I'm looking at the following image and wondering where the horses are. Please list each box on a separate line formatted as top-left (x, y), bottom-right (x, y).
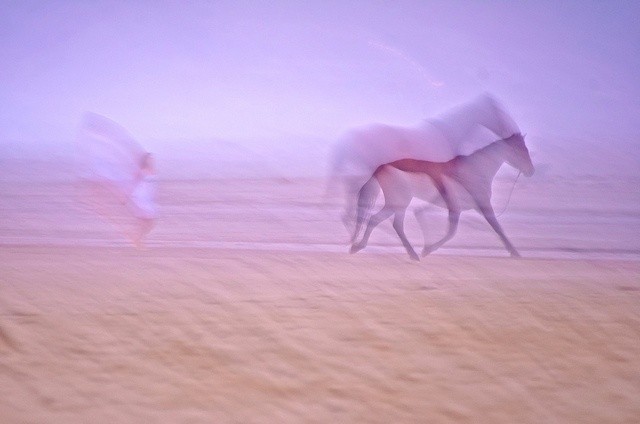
top-left (324, 93), bottom-right (521, 246)
top-left (347, 132), bottom-right (535, 261)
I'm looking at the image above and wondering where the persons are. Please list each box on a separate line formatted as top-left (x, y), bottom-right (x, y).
top-left (128, 152), bottom-right (164, 247)
